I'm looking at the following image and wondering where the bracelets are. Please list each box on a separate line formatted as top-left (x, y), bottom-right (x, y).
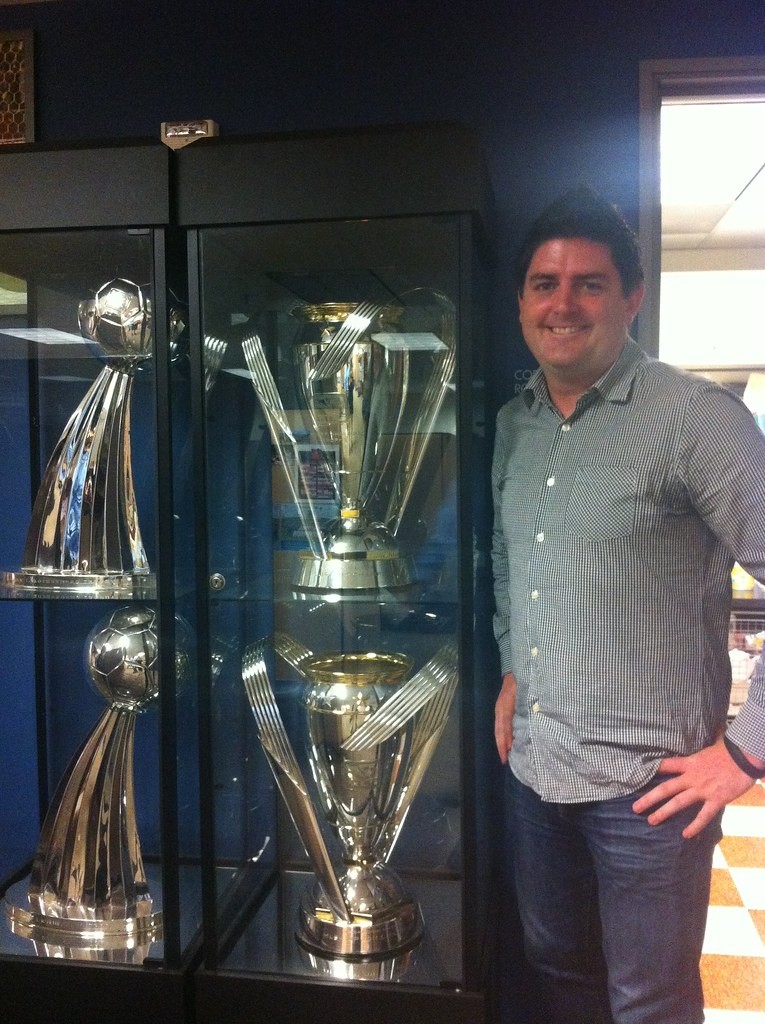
top-left (722, 735), bottom-right (765, 781)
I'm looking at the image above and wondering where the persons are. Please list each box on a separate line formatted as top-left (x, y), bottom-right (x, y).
top-left (492, 189), bottom-right (765, 1023)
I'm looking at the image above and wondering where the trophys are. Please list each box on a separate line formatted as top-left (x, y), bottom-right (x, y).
top-left (3, 274), bottom-right (164, 593)
top-left (242, 633), bottom-right (462, 963)
top-left (240, 287), bottom-right (462, 593)
top-left (3, 603), bottom-right (167, 934)
